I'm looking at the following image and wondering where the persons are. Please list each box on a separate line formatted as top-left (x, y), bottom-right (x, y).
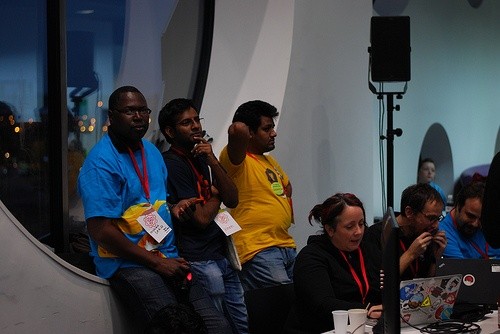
top-left (77, 85), bottom-right (203, 334)
top-left (158, 101), bottom-right (251, 334)
top-left (218, 99), bottom-right (297, 285)
top-left (419, 158), bottom-right (448, 204)
top-left (289, 153), bottom-right (500, 334)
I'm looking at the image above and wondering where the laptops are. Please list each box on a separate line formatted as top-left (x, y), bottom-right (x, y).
top-left (435, 258), bottom-right (500, 306)
top-left (364, 274), bottom-right (463, 328)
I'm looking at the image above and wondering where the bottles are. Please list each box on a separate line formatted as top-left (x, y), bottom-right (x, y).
top-left (446, 194), bottom-right (455, 215)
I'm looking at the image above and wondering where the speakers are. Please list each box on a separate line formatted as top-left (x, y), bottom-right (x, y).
top-left (369, 15), bottom-right (411, 82)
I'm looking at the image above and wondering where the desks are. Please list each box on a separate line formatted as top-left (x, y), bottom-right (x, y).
top-left (322, 309), bottom-right (500, 334)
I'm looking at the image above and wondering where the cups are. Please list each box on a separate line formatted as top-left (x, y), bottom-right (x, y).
top-left (349, 309), bottom-right (368, 334)
top-left (332, 310), bottom-right (349, 334)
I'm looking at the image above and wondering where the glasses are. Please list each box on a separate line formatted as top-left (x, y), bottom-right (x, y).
top-left (180, 118), bottom-right (205, 127)
top-left (115, 108), bottom-right (151, 116)
top-left (420, 211), bottom-right (446, 223)
